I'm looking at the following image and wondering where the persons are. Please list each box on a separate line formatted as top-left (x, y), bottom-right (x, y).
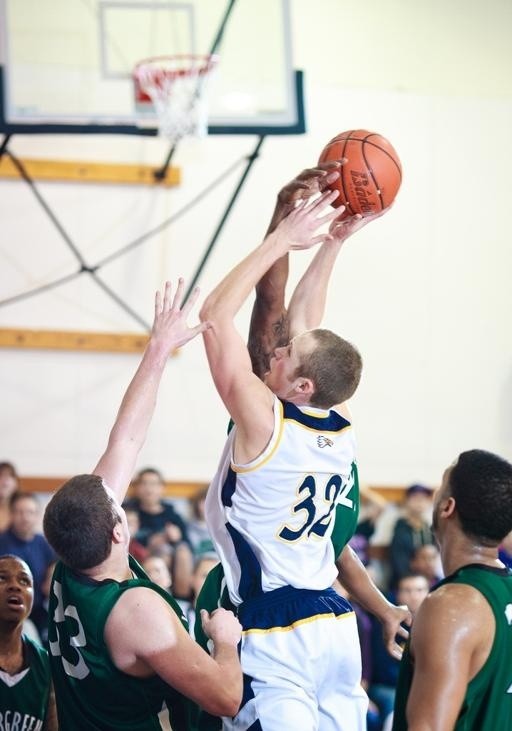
top-left (164, 158), bottom-right (416, 731)
top-left (199, 187), bottom-right (399, 730)
top-left (2, 446), bottom-right (512, 731)
top-left (42, 276), bottom-right (246, 731)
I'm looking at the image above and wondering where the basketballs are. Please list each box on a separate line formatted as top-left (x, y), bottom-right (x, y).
top-left (317, 129), bottom-right (403, 219)
